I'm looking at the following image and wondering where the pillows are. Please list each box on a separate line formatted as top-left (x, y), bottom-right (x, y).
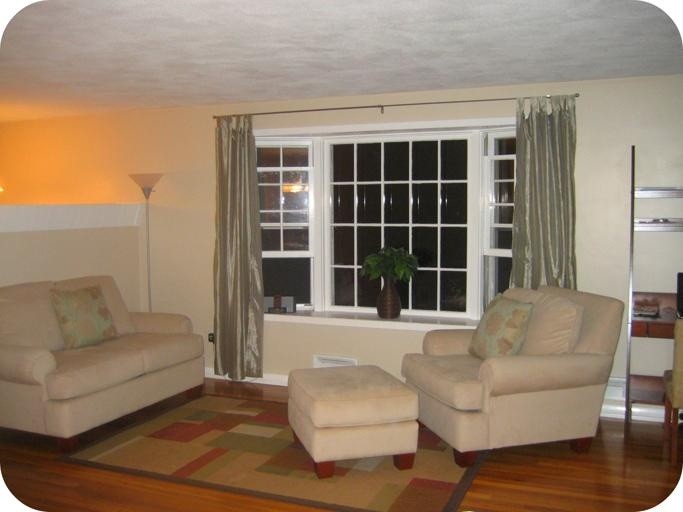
top-left (51, 284), bottom-right (120, 350)
top-left (468, 293), bottom-right (534, 363)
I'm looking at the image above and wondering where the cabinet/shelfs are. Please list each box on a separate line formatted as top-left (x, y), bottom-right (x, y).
top-left (624, 142), bottom-right (683, 437)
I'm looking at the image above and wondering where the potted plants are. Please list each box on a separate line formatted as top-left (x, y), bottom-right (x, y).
top-left (362, 251), bottom-right (419, 318)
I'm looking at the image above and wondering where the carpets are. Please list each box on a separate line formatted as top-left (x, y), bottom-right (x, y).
top-left (71, 394), bottom-right (486, 511)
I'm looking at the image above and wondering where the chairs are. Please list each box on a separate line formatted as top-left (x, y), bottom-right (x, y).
top-left (401, 285), bottom-right (626, 468)
top-left (663, 321), bottom-right (683, 462)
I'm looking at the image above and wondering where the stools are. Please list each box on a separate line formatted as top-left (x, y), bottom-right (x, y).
top-left (285, 365), bottom-right (417, 476)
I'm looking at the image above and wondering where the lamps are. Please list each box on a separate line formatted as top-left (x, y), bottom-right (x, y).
top-left (129, 172), bottom-right (162, 311)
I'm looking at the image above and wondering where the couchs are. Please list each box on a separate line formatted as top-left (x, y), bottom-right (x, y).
top-left (2, 276), bottom-right (206, 465)
